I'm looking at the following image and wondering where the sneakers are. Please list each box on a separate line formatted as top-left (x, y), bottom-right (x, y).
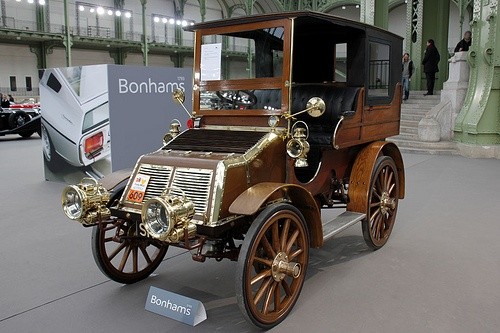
top-left (403, 95), bottom-right (409, 100)
top-left (423, 91), bottom-right (434, 95)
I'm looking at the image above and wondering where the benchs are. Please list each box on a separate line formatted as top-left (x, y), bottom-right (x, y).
top-left (246, 83), bottom-right (365, 149)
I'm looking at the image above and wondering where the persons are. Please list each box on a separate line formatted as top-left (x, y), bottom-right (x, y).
top-left (454, 31), bottom-right (472, 52)
top-left (0, 93), bottom-right (14, 108)
top-left (422, 39), bottom-right (440, 95)
top-left (402, 53), bottom-right (413, 101)
